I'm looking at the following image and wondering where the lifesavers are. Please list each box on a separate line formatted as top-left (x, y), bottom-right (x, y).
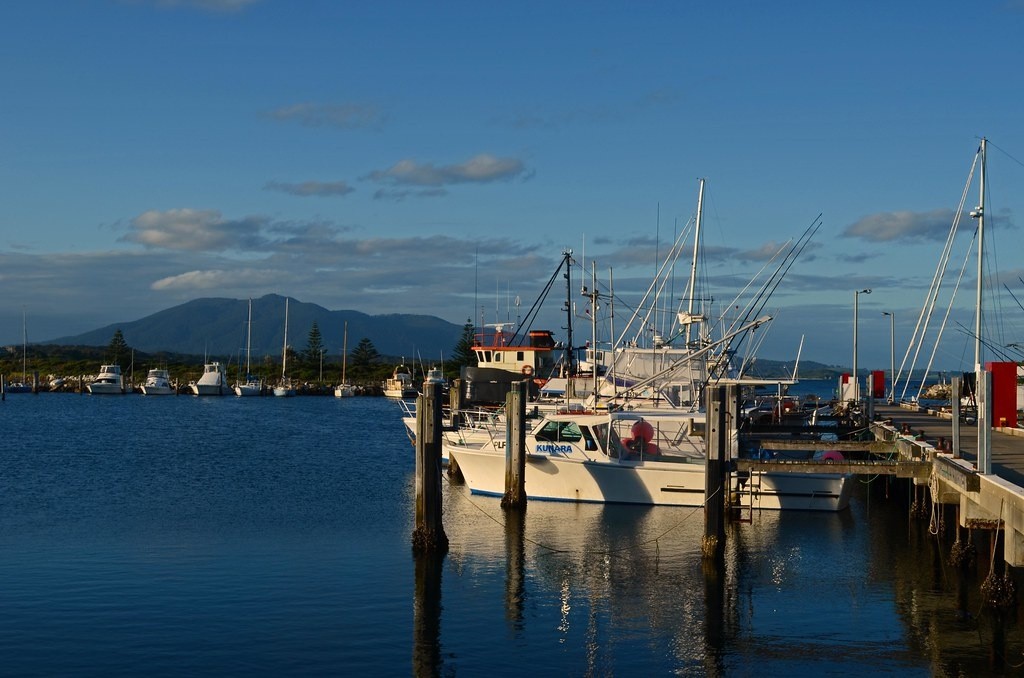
top-left (522, 365), bottom-right (534, 375)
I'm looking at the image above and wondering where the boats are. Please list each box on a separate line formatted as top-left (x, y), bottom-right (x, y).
top-left (90, 364), bottom-right (124, 393)
top-left (140, 369), bottom-right (175, 395)
top-left (194, 363), bottom-right (229, 394)
top-left (382, 356), bottom-right (419, 399)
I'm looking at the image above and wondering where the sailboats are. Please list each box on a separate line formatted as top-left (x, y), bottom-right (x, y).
top-left (401, 129), bottom-right (1024, 513)
top-left (331, 320), bottom-right (357, 398)
top-left (234, 297), bottom-right (268, 396)
top-left (274, 298), bottom-right (297, 397)
